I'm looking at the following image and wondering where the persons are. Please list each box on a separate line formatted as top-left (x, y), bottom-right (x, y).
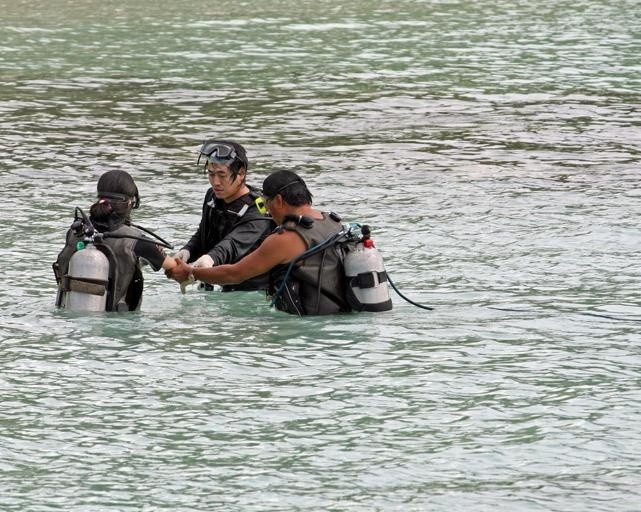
top-left (53, 169), bottom-right (179, 311)
top-left (164, 141), bottom-right (276, 292)
top-left (164, 169), bottom-right (352, 315)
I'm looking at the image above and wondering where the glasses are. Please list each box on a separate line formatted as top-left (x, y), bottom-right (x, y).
top-left (199, 141), bottom-right (244, 164)
top-left (131, 195), bottom-right (141, 210)
top-left (255, 195), bottom-right (270, 218)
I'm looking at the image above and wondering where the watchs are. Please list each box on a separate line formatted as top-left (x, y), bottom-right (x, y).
top-left (187, 266), bottom-right (197, 282)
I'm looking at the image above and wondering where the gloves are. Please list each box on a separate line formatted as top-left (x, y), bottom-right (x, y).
top-left (186, 253), bottom-right (215, 286)
top-left (164, 248), bottom-right (191, 279)
